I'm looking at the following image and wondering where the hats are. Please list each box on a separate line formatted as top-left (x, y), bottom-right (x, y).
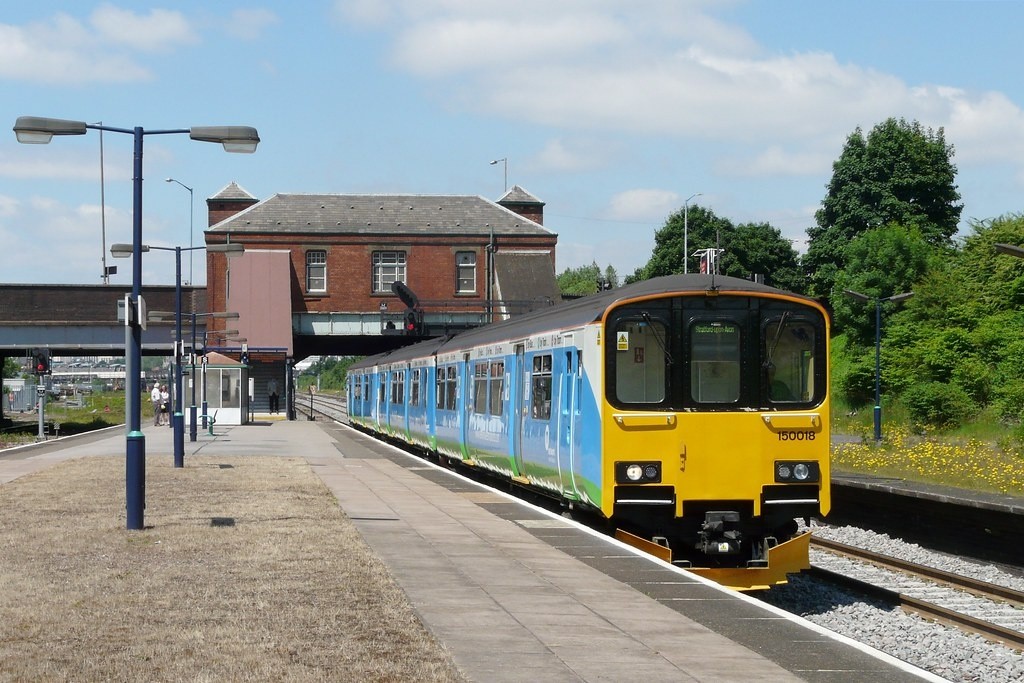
top-left (154, 383), bottom-right (160, 388)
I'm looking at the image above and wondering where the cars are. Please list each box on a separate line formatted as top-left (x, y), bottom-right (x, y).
top-left (23, 359), bottom-right (146, 403)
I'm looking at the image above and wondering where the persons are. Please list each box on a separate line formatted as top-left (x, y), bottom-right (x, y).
top-left (160, 385), bottom-right (170, 425)
top-left (151, 383), bottom-right (162, 426)
top-left (769, 362), bottom-right (794, 400)
top-left (268, 376), bottom-right (280, 415)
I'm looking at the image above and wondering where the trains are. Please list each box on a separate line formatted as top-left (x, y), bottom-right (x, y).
top-left (346, 269), bottom-right (832, 594)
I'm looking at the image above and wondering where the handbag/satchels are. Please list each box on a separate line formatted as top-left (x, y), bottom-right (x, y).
top-left (161, 405), bottom-right (166, 409)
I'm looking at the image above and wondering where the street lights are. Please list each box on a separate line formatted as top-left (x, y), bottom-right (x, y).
top-left (490, 158), bottom-right (508, 194)
top-left (847, 290), bottom-right (916, 441)
top-left (12, 115), bottom-right (262, 530)
top-left (148, 311), bottom-right (242, 442)
top-left (109, 243), bottom-right (247, 469)
top-left (191, 335), bottom-right (248, 355)
top-left (166, 177), bottom-right (194, 285)
top-left (683, 193), bottom-right (706, 274)
top-left (170, 329), bottom-right (240, 432)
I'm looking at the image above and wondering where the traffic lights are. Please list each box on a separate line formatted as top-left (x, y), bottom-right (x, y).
top-left (404, 309), bottom-right (420, 337)
top-left (32, 347), bottom-right (50, 374)
